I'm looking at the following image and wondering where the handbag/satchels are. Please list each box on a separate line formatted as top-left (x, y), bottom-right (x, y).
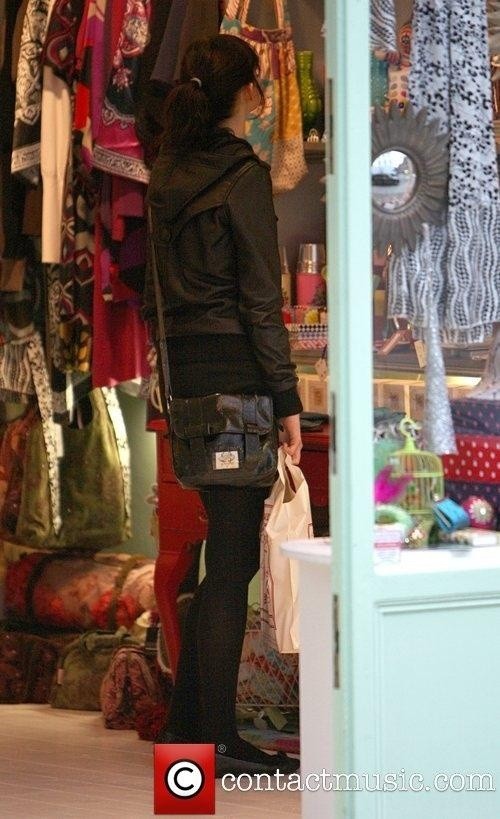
top-left (1, 619), bottom-right (170, 728)
top-left (2, 408), bottom-right (132, 560)
top-left (216, 17), bottom-right (310, 195)
top-left (164, 394), bottom-right (278, 489)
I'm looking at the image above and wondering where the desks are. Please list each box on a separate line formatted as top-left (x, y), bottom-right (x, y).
top-left (279, 528), bottom-right (500, 819)
top-left (146, 418), bottom-right (328, 686)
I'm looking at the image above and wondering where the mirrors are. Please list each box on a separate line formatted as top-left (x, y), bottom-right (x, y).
top-left (372, 98), bottom-right (450, 258)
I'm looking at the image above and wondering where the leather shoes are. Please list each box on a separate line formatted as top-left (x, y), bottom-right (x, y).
top-left (153, 731), bottom-right (301, 779)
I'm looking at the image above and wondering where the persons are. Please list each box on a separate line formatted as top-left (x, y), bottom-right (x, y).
top-left (144, 34), bottom-right (303, 777)
